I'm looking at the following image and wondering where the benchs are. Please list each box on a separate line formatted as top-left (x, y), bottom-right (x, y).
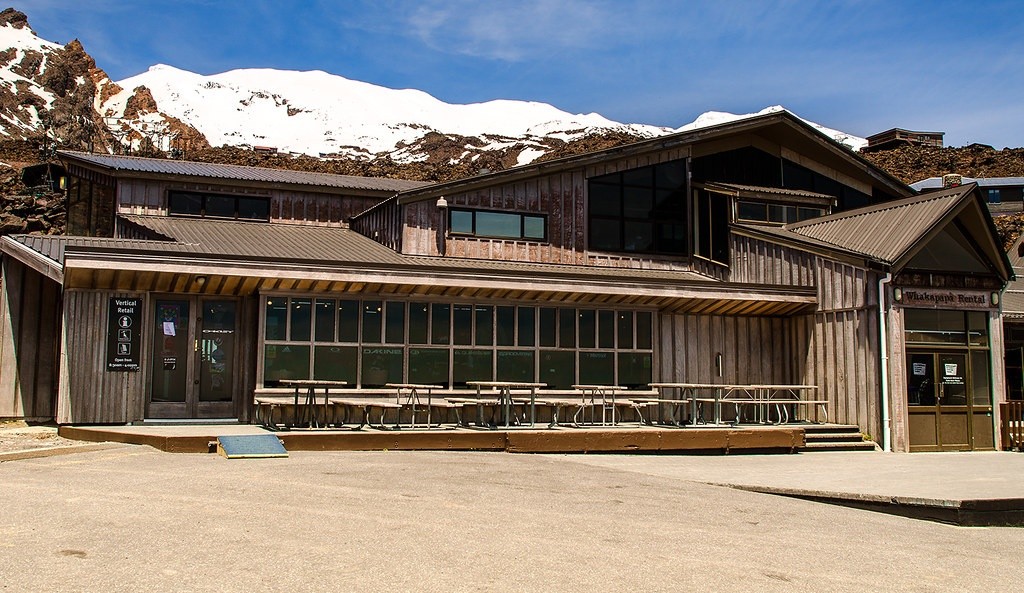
top-left (254, 395), bottom-right (830, 427)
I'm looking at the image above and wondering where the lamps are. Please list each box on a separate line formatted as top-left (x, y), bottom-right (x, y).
top-left (893, 287), bottom-right (902, 301)
top-left (991, 292), bottom-right (999, 305)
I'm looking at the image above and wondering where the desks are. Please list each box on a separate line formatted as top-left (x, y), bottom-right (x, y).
top-left (279, 379), bottom-right (347, 432)
top-left (386, 383), bottom-right (442, 429)
top-left (752, 384), bottom-right (819, 423)
top-left (647, 382), bottom-right (718, 429)
top-left (467, 381), bottom-right (547, 427)
top-left (705, 383), bottom-right (755, 422)
top-left (573, 385), bottom-right (627, 427)
